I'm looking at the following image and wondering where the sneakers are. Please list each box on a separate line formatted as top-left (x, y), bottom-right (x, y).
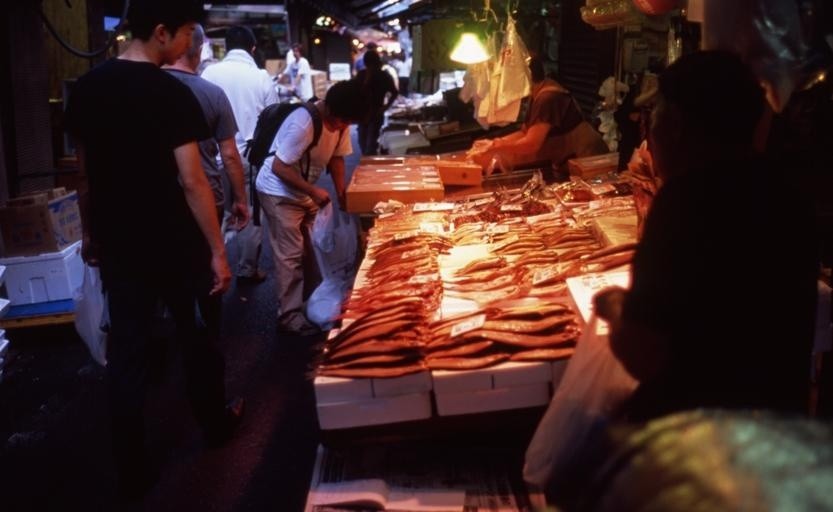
top-left (278, 311), bottom-right (321, 336)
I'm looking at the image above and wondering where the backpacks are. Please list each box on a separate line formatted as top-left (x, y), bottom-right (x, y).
top-left (248, 102), bottom-right (344, 168)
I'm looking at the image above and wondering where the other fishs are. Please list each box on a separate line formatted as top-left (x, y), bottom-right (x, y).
top-left (305, 212), bottom-right (639, 378)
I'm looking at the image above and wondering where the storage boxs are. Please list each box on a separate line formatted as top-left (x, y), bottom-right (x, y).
top-left (1, 190), bottom-right (83, 257)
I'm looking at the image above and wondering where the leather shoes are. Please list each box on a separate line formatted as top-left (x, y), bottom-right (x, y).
top-left (237, 272), bottom-right (267, 288)
top-left (205, 397), bottom-right (246, 447)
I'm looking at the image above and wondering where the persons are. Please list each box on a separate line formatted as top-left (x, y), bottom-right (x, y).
top-left (471, 50), bottom-right (609, 179)
top-left (254, 77), bottom-right (367, 338)
top-left (348, 41), bottom-right (412, 98)
top-left (274, 40), bottom-right (315, 101)
top-left (203, 25), bottom-right (282, 288)
top-left (352, 51), bottom-right (399, 157)
top-left (592, 49), bottom-right (819, 419)
top-left (60, 1), bottom-right (247, 502)
top-left (159, 20), bottom-right (252, 236)
